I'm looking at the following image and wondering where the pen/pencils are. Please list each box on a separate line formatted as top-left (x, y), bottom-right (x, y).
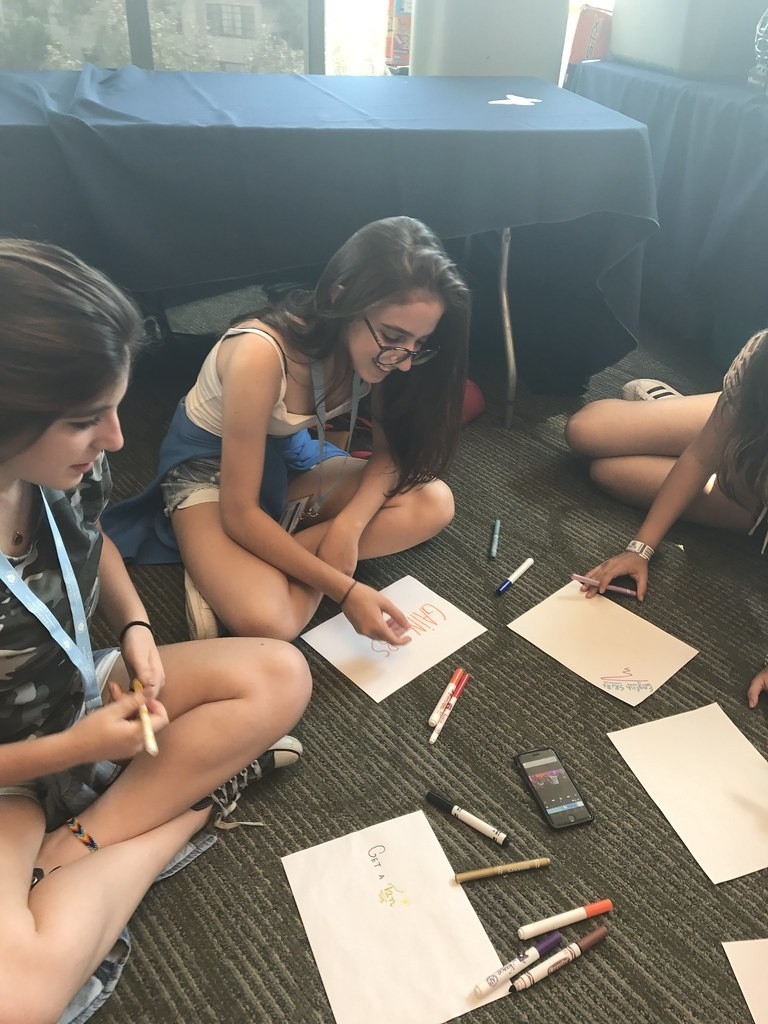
top-left (490, 519), bottom-right (500, 558)
top-left (133, 679), bottom-right (159, 755)
top-left (429, 673), bottom-right (470, 745)
top-left (455, 858), bottom-right (551, 883)
top-left (571, 574), bottom-right (636, 597)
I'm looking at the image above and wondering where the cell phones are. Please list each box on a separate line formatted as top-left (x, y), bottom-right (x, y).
top-left (515, 748), bottom-right (593, 830)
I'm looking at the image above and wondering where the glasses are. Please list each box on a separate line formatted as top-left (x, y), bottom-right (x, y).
top-left (361, 311), bottom-right (441, 366)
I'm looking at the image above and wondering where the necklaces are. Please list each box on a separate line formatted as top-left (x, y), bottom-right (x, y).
top-left (1, 492), bottom-right (33, 545)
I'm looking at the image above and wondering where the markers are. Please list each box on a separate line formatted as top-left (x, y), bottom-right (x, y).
top-left (475, 932), bottom-right (562, 997)
top-left (509, 925), bottom-right (609, 994)
top-left (427, 668), bottom-right (463, 727)
top-left (497, 557), bottom-right (534, 592)
top-left (426, 791), bottom-right (510, 846)
top-left (517, 897), bottom-right (614, 943)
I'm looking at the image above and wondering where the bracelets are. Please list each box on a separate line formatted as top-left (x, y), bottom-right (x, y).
top-left (118, 620), bottom-right (155, 641)
top-left (336, 580), bottom-right (357, 607)
top-left (625, 539), bottom-right (654, 560)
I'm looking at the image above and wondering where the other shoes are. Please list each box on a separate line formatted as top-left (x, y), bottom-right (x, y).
top-left (184, 567), bottom-right (218, 641)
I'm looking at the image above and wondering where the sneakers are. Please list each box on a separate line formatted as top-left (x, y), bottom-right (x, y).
top-left (622, 378), bottom-right (685, 401)
top-left (29, 865), bottom-right (61, 891)
top-left (189, 734), bottom-right (303, 830)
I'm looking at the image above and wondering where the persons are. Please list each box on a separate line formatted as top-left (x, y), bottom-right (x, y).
top-left (98, 215), bottom-right (471, 641)
top-left (0, 236), bottom-right (311, 1024)
top-left (566, 330), bottom-right (768, 708)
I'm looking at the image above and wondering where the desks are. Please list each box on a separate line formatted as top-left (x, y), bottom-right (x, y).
top-left (567, 56), bottom-right (766, 383)
top-left (0, 65), bottom-right (659, 427)
